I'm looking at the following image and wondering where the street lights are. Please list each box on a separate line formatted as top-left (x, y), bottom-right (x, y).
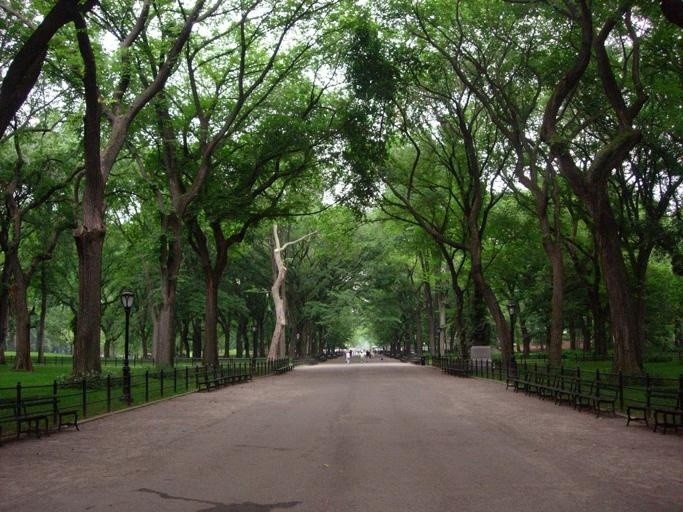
top-left (507, 303), bottom-right (515, 383)
top-left (119, 290), bottom-right (135, 402)
top-left (250, 325), bottom-right (258, 370)
top-left (436, 325), bottom-right (442, 359)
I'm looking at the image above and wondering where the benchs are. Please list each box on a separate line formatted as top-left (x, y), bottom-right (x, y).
top-left (195, 357), bottom-right (293, 392)
top-left (444, 359), bottom-right (469, 378)
top-left (504, 368), bottom-right (682, 434)
top-left (2, 398), bottom-right (80, 440)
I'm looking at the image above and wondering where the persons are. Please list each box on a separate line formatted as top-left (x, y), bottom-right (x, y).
top-left (345, 349), bottom-right (371, 364)
